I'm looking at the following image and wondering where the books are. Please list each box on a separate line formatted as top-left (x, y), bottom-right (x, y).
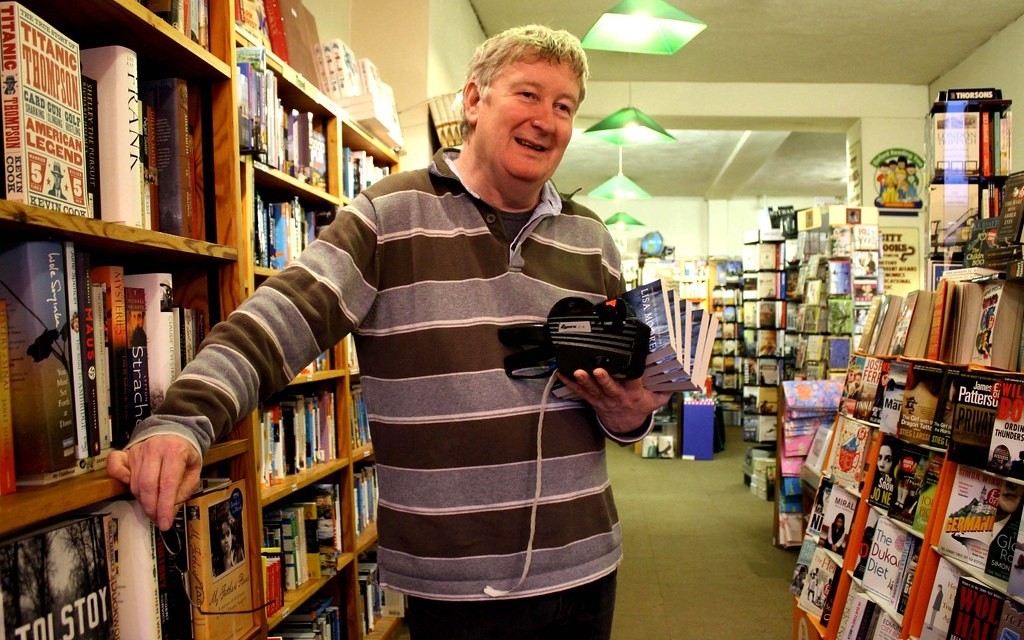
top-left (641, 206), bottom-right (880, 548)
top-left (789, 87), bottom-right (1024, 640)
top-left (549, 279), bottom-right (719, 400)
top-left (428, 90), bottom-right (467, 148)
top-left (0, 0), bottom-right (406, 640)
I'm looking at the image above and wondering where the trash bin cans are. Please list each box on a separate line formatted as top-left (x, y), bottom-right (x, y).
top-left (681, 399), bottom-right (717, 460)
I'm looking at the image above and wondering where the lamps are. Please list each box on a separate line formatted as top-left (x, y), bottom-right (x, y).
top-left (584, 106), bottom-right (677, 146)
top-left (587, 174), bottom-right (653, 231)
top-left (581, 0), bottom-right (708, 56)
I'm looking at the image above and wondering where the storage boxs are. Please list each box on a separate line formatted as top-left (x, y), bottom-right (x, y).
top-left (635, 435), bottom-right (675, 458)
top-left (682, 401), bottom-right (715, 458)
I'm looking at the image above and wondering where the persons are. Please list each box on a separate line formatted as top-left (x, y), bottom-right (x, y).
top-left (105, 24), bottom-right (671, 640)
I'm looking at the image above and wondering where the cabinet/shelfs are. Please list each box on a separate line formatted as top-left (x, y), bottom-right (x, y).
top-left (641, 87), bottom-right (1024, 640)
top-left (0, 0), bottom-right (407, 640)
